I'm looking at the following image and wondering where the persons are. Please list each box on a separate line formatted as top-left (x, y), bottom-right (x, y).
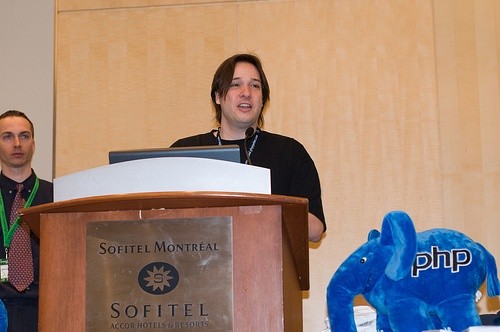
top-left (1, 110), bottom-right (55, 332)
top-left (168, 54), bottom-right (328, 244)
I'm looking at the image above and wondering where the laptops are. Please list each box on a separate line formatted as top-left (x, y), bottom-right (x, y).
top-left (108, 145), bottom-right (241, 164)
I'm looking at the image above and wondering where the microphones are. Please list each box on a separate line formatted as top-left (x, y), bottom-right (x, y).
top-left (243, 127), bottom-right (255, 165)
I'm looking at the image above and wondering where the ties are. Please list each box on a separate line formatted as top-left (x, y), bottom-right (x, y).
top-left (6, 184), bottom-right (34, 292)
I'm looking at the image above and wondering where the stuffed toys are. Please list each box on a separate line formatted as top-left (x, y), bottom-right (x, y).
top-left (325, 208), bottom-right (500, 332)
